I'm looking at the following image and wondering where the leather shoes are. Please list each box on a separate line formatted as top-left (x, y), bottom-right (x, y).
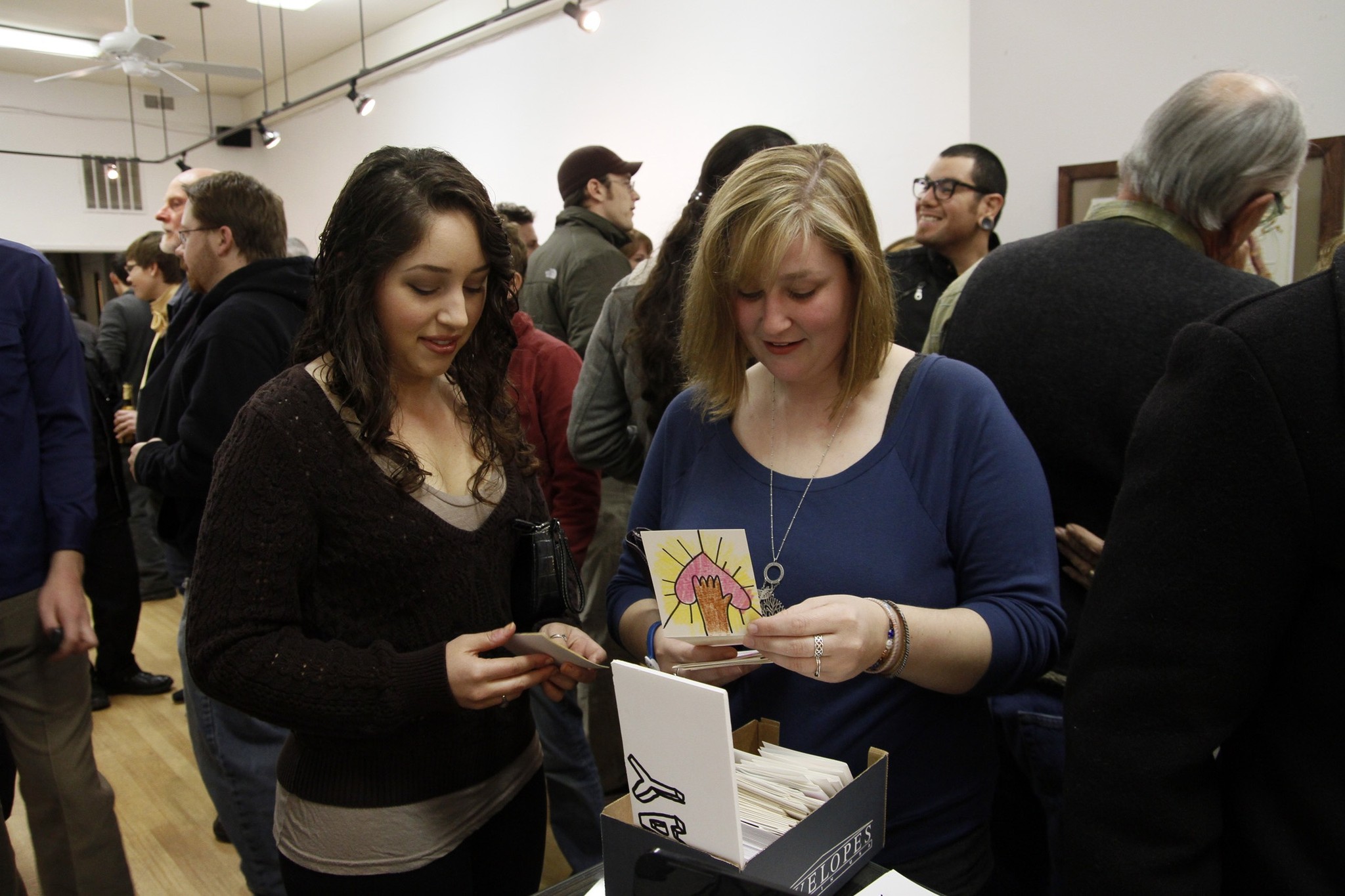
top-left (88, 670), bottom-right (173, 711)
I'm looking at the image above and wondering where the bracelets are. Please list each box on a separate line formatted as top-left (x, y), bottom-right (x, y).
top-left (863, 597), bottom-right (911, 680)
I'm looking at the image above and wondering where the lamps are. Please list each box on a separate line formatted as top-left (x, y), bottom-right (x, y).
top-left (176, 159), bottom-right (191, 172)
top-left (346, 89), bottom-right (375, 116)
top-left (563, 2), bottom-right (602, 33)
top-left (106, 164), bottom-right (119, 179)
top-left (257, 128), bottom-right (281, 151)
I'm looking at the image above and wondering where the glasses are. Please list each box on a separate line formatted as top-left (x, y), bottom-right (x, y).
top-left (608, 177), bottom-right (635, 194)
top-left (178, 227), bottom-right (208, 245)
top-left (124, 264), bottom-right (138, 272)
top-left (912, 175), bottom-right (984, 201)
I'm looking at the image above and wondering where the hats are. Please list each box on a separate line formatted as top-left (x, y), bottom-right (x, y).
top-left (556, 146), bottom-right (643, 201)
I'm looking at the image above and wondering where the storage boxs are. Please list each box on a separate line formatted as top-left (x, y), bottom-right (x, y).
top-left (599, 717), bottom-right (889, 896)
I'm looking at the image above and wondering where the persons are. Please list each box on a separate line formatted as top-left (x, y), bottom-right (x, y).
top-left (112, 230), bottom-right (187, 445)
top-left (570, 125), bottom-right (796, 485)
top-left (879, 145), bottom-right (1007, 352)
top-left (491, 203), bottom-right (538, 262)
top-left (58, 277), bottom-right (172, 711)
top-left (96, 258), bottom-right (156, 382)
top-left (619, 228), bottom-right (655, 269)
top-left (132, 170), bottom-right (321, 843)
top-left (1139, 245), bottom-right (1344, 894)
top-left (930, 66), bottom-right (1308, 689)
top-left (490, 213), bottom-right (615, 878)
top-left (2, 239), bottom-right (137, 896)
top-left (134, 168), bottom-right (220, 442)
top-left (64, 296), bottom-right (96, 366)
top-left (609, 143), bottom-right (1064, 890)
top-left (518, 144), bottom-right (653, 800)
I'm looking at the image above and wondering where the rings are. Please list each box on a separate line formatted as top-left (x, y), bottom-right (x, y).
top-left (814, 656), bottom-right (820, 678)
top-left (502, 696), bottom-right (507, 703)
top-left (196, 144), bottom-right (606, 896)
top-left (815, 634), bottom-right (823, 660)
top-left (550, 634), bottom-right (567, 642)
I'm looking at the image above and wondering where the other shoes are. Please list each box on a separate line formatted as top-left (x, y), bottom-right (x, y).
top-left (137, 580), bottom-right (179, 602)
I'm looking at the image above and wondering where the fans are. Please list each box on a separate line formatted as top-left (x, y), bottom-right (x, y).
top-left (33, 1), bottom-right (264, 96)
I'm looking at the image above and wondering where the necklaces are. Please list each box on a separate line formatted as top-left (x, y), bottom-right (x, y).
top-left (763, 375), bottom-right (854, 583)
